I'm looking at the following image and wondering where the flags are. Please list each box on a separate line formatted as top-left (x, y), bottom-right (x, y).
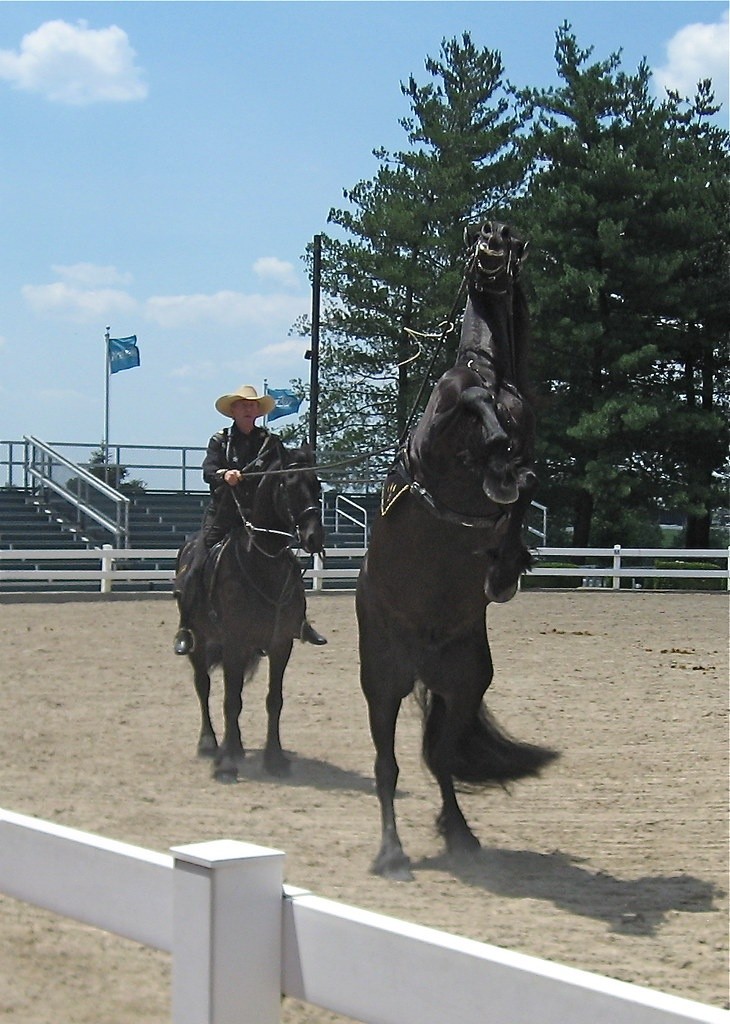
top-left (109, 336), bottom-right (140, 374)
top-left (267, 388), bottom-right (304, 420)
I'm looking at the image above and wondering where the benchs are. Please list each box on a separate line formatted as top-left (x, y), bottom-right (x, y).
top-left (0, 482), bottom-right (382, 591)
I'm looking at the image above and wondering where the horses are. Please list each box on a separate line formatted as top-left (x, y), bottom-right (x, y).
top-left (353, 222), bottom-right (566, 882)
top-left (170, 432), bottom-right (327, 785)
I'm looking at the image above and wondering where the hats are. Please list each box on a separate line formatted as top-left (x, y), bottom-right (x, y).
top-left (214, 386), bottom-right (276, 419)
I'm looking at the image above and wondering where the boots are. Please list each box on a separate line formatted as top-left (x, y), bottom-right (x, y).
top-left (296, 620), bottom-right (328, 646)
top-left (173, 590), bottom-right (195, 654)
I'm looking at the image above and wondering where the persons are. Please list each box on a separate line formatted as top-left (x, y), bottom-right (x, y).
top-left (174, 385), bottom-right (328, 655)
